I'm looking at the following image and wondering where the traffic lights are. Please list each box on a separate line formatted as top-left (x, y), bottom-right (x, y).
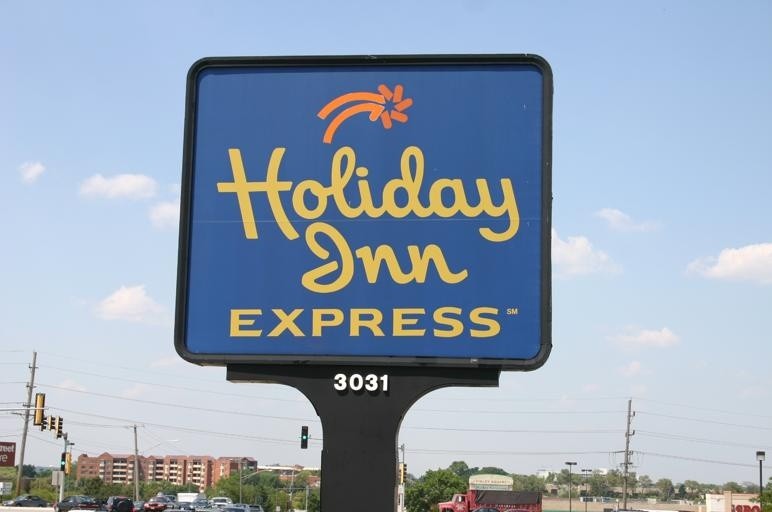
top-left (300, 425), bottom-right (309, 450)
top-left (60, 453), bottom-right (66, 471)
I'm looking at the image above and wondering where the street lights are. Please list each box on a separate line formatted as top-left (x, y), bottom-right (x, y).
top-left (581, 469), bottom-right (592, 512)
top-left (755, 450), bottom-right (766, 508)
top-left (565, 461), bottom-right (577, 511)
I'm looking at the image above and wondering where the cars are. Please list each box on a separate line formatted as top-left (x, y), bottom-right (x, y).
top-left (102, 495), bottom-right (135, 512)
top-left (134, 492), bottom-right (265, 512)
top-left (2, 494), bottom-right (48, 507)
top-left (53, 495), bottom-right (101, 512)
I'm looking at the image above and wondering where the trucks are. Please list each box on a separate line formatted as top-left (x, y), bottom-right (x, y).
top-left (438, 489), bottom-right (543, 512)
